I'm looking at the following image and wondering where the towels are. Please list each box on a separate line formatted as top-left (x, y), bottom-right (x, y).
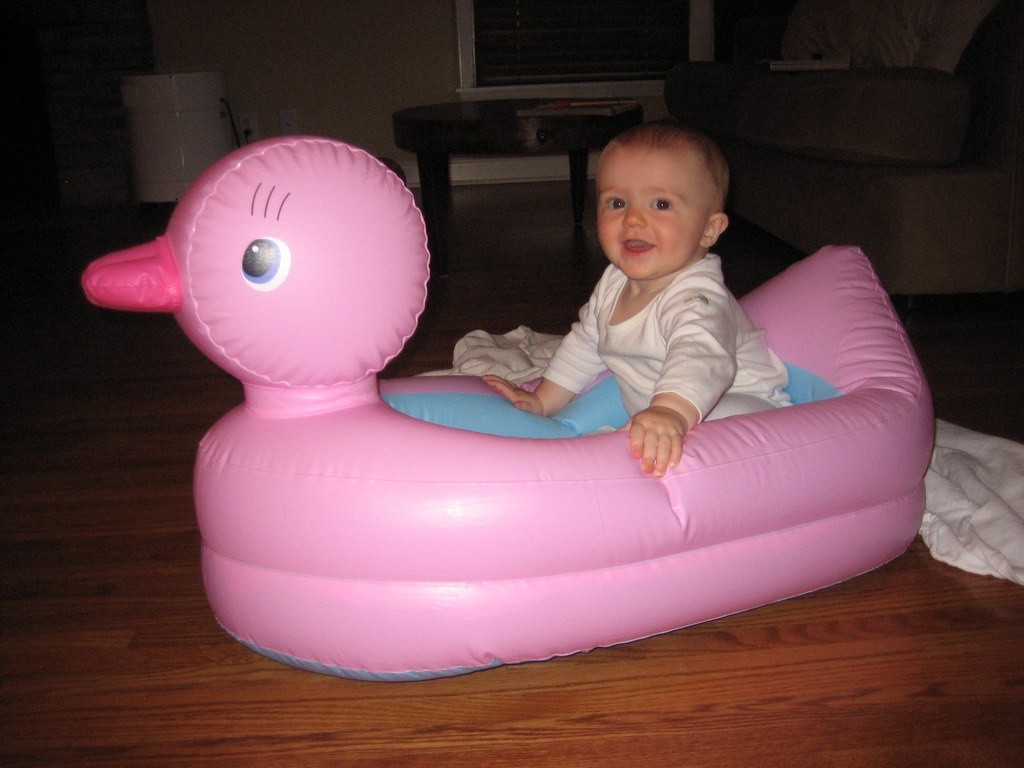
top-left (414, 324), bottom-right (569, 389)
top-left (918, 418), bottom-right (1024, 586)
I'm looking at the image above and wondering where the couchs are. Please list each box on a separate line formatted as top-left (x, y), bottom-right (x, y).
top-left (659, 0), bottom-right (1024, 312)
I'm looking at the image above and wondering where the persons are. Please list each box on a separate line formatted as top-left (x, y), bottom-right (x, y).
top-left (480, 117), bottom-right (794, 477)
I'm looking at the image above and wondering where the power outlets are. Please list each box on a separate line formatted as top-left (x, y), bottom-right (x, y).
top-left (279, 112), bottom-right (296, 135)
top-left (238, 113), bottom-right (258, 141)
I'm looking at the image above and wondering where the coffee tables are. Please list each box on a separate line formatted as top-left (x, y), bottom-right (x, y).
top-left (392, 95), bottom-right (641, 273)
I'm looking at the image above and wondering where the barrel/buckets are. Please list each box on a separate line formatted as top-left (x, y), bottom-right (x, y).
top-left (120, 67), bottom-right (239, 207)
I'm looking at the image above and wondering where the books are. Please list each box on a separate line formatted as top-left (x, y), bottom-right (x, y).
top-left (771, 55), bottom-right (873, 70)
top-left (516, 99), bottom-right (638, 117)
